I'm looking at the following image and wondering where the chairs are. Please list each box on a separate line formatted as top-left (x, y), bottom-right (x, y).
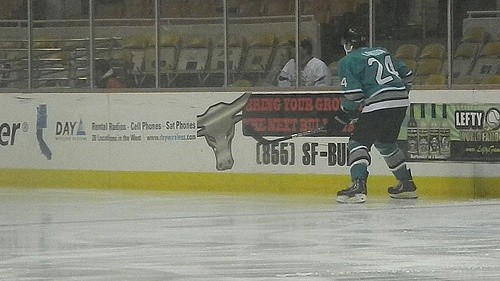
top-left (0, 29), bottom-right (316, 86)
top-left (397, 27), bottom-right (500, 85)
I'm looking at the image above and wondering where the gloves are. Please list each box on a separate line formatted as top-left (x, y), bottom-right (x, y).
top-left (325, 108), bottom-right (348, 134)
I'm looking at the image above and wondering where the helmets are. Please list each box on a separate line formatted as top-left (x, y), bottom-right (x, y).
top-left (340, 27), bottom-right (366, 55)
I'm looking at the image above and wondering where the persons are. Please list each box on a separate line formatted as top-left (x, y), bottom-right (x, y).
top-left (278, 38), bottom-right (332, 87)
top-left (324, 27), bottom-right (418, 203)
top-left (93, 59), bottom-right (121, 88)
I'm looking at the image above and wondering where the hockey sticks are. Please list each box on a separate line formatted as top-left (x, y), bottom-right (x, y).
top-left (244, 123), bottom-right (326, 145)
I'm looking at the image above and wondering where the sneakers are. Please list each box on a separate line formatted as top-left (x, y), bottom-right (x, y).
top-left (336, 178), bottom-right (368, 204)
top-left (387, 180), bottom-right (418, 198)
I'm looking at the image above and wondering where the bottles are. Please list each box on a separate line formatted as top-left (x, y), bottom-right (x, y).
top-left (429, 104), bottom-right (440, 160)
top-left (440, 104), bottom-right (451, 159)
top-left (406, 103), bottom-right (419, 160)
top-left (417, 103), bottom-right (430, 160)
